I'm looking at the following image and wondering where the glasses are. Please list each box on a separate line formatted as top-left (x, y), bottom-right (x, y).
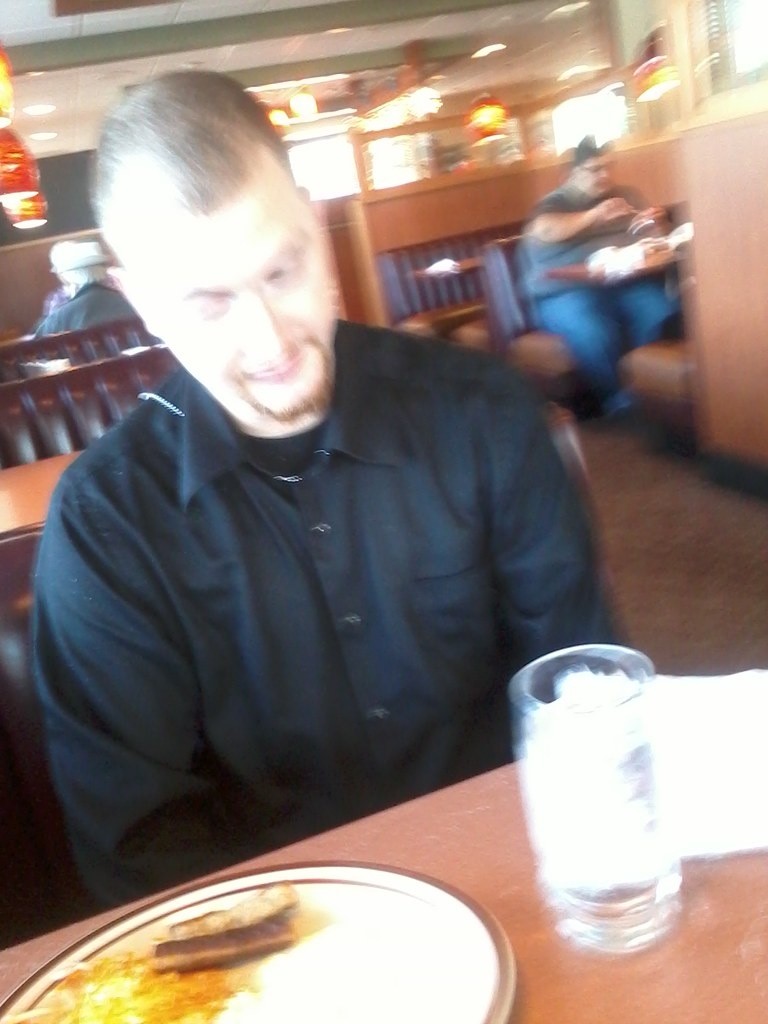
top-left (581, 164), bottom-right (604, 172)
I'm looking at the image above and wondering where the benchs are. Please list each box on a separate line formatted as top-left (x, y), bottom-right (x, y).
top-left (378, 238), bottom-right (696, 440)
top-left (0, 317), bottom-right (184, 953)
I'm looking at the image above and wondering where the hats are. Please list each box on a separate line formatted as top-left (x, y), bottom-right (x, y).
top-left (55, 241), bottom-right (110, 273)
top-left (575, 135), bottom-right (616, 164)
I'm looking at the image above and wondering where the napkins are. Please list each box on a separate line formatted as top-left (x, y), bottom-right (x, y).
top-left (563, 668), bottom-right (768, 861)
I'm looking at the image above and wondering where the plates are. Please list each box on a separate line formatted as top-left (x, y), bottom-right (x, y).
top-left (0, 860), bottom-right (515, 1024)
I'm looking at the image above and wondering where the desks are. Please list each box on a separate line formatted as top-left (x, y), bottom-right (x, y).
top-left (548, 244), bottom-right (678, 289)
top-left (414, 254), bottom-right (483, 277)
top-left (0, 762), bottom-right (768, 1024)
top-left (0, 451), bottom-right (86, 539)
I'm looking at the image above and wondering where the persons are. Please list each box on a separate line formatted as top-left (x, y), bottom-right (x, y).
top-left (30, 71), bottom-right (617, 918)
top-left (32, 239), bottom-right (146, 369)
top-left (518, 143), bottom-right (681, 418)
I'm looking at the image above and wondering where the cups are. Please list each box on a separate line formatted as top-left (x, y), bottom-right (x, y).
top-left (507, 642), bottom-right (685, 958)
